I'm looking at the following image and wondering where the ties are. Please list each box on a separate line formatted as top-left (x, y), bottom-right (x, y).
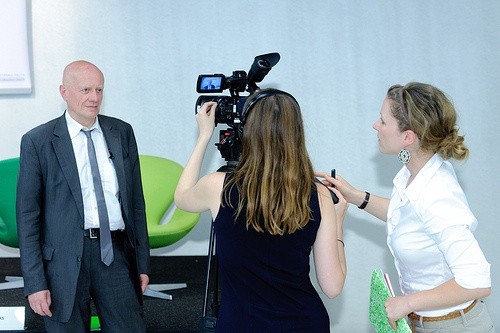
top-left (81, 128), bottom-right (114, 266)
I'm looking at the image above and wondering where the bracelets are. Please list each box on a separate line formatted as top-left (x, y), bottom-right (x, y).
top-left (358, 191), bottom-right (370, 209)
top-left (337, 238), bottom-right (346, 248)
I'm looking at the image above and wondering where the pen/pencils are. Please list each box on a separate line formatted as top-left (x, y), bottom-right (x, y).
top-left (330, 169), bottom-right (336, 189)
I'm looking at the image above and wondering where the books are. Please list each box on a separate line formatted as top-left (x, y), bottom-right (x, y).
top-left (369, 270), bottom-right (412, 333)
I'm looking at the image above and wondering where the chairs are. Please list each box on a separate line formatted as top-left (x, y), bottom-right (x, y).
top-left (0, 155), bottom-right (200, 300)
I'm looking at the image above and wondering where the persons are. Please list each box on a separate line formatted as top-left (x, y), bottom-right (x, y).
top-left (15, 60), bottom-right (150, 333)
top-left (204, 79), bottom-right (215, 90)
top-left (174, 88), bottom-right (349, 333)
top-left (314, 81), bottom-right (497, 333)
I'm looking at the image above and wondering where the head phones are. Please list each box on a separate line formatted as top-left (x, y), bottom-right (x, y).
top-left (237, 89), bottom-right (300, 140)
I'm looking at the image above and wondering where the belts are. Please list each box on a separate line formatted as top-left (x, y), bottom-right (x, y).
top-left (409, 299), bottom-right (478, 333)
top-left (82, 227), bottom-right (100, 238)
